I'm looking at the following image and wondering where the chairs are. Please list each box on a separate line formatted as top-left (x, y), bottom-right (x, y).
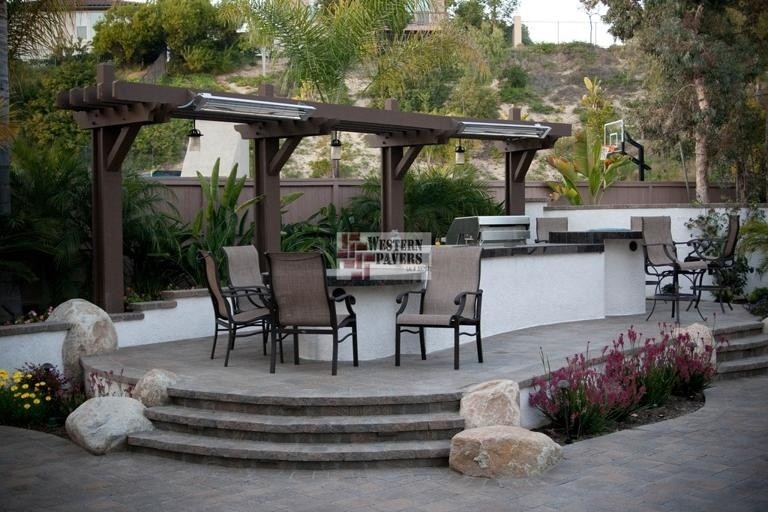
top-left (198, 243), bottom-right (485, 376)
top-left (534, 213), bottom-right (741, 327)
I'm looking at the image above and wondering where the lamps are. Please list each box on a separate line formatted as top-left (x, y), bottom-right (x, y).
top-left (455, 135), bottom-right (465, 165)
top-left (189, 118), bottom-right (203, 152)
top-left (330, 129), bottom-right (342, 160)
